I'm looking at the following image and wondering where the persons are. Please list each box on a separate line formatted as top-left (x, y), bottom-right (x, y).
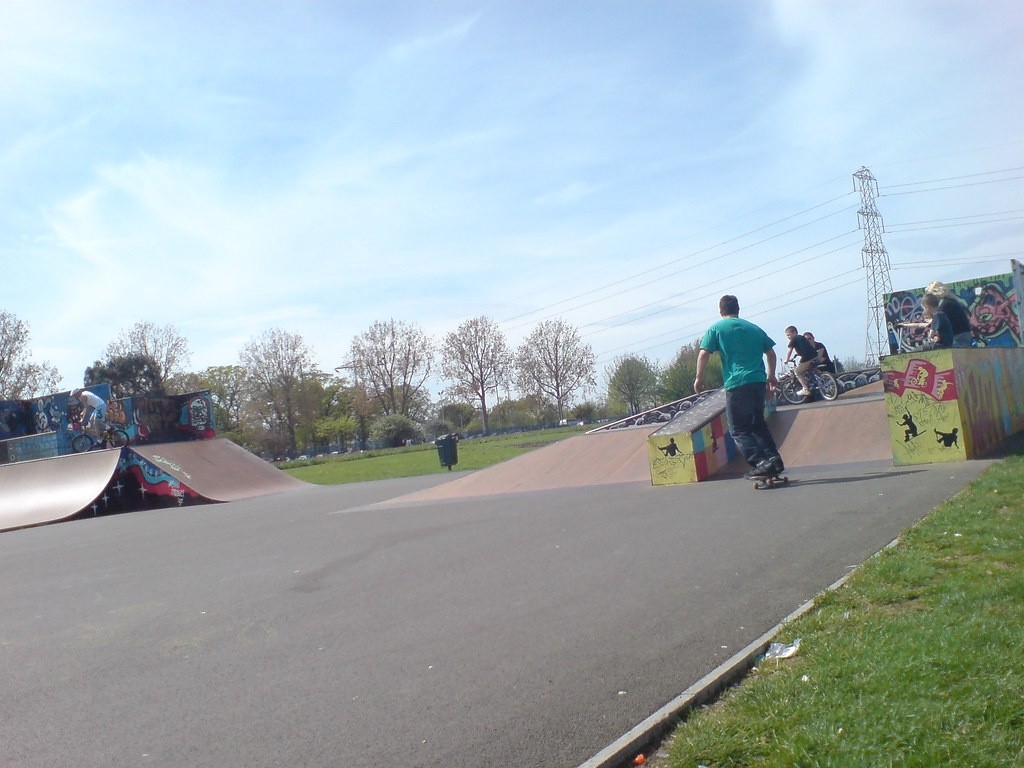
top-left (913, 280), bottom-right (972, 351)
top-left (694, 295), bottom-right (785, 479)
top-left (70, 387), bottom-right (116, 447)
top-left (785, 326), bottom-right (817, 395)
top-left (803, 331), bottom-right (833, 373)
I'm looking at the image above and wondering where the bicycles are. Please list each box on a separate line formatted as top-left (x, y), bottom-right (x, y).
top-left (70, 420), bottom-right (129, 453)
top-left (781, 360), bottom-right (838, 404)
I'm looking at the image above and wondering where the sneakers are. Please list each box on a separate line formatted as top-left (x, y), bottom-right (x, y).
top-left (797, 388), bottom-right (811, 396)
top-left (744, 460), bottom-right (775, 479)
top-left (769, 456), bottom-right (785, 473)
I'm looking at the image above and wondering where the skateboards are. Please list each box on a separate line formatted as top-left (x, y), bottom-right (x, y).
top-left (749, 474), bottom-right (789, 490)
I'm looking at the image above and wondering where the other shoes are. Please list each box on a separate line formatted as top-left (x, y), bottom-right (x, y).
top-left (106, 425), bottom-right (115, 433)
top-left (92, 442), bottom-right (104, 447)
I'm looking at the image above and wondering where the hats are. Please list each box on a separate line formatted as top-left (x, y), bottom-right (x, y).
top-left (70, 387), bottom-right (79, 396)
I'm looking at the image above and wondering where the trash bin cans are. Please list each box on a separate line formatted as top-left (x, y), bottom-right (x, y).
top-left (435, 434), bottom-right (458, 466)
